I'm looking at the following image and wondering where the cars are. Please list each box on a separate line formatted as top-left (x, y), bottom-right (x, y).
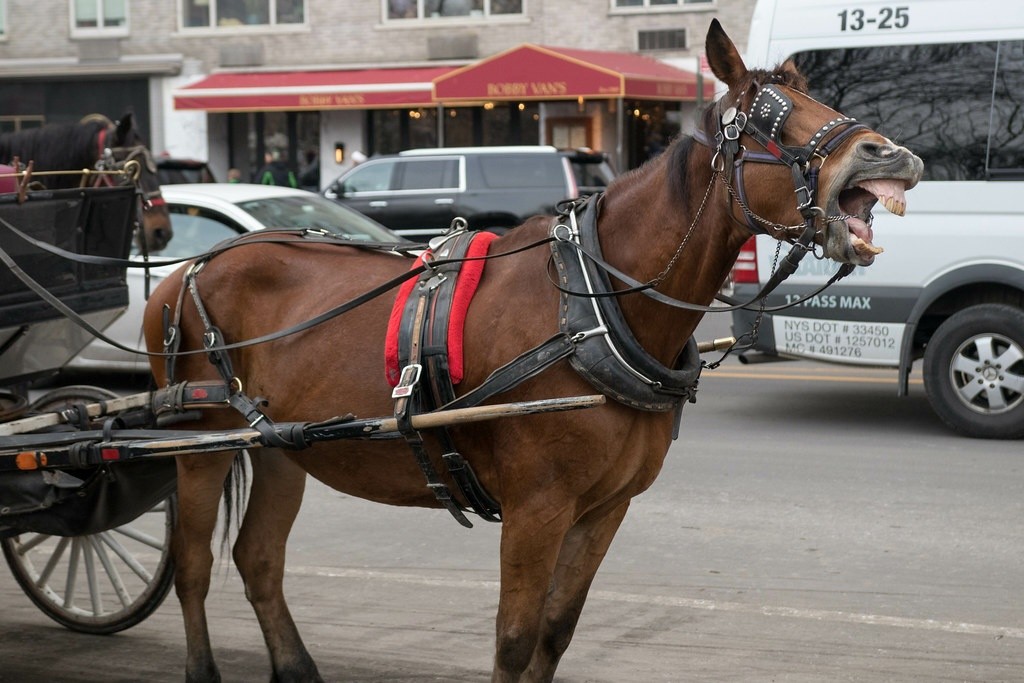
top-left (35, 175), bottom-right (436, 514)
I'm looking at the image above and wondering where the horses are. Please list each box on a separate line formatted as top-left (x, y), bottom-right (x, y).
top-left (140, 19), bottom-right (923, 683)
top-left (0, 112), bottom-right (172, 257)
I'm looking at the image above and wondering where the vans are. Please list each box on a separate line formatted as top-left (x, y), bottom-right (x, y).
top-left (721, 0), bottom-right (1023, 439)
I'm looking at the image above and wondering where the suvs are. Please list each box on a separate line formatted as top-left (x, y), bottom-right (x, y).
top-left (313, 131), bottom-right (618, 261)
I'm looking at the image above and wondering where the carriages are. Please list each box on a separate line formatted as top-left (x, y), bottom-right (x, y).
top-left (16, 2), bottom-right (925, 683)
